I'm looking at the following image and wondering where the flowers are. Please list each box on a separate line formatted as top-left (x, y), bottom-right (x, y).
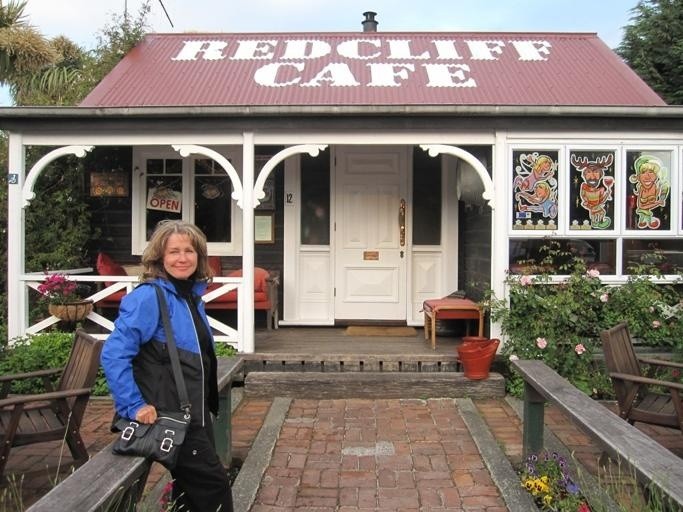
top-left (38, 274), bottom-right (80, 303)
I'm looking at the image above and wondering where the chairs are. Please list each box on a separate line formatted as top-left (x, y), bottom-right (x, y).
top-left (0, 328), bottom-right (104, 469)
top-left (600, 322), bottom-right (683, 468)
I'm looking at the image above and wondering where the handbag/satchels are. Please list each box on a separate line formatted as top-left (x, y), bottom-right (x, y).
top-left (112, 409), bottom-right (191, 469)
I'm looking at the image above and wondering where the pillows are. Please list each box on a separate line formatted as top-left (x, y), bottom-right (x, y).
top-left (97, 252), bottom-right (269, 291)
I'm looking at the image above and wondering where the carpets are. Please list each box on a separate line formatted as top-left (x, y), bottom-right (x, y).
top-left (345, 326), bottom-right (419, 336)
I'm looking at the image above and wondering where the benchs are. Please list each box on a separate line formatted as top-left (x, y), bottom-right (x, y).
top-left (422, 296), bottom-right (483, 351)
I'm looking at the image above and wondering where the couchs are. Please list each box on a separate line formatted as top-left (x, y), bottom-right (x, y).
top-left (94, 264), bottom-right (280, 332)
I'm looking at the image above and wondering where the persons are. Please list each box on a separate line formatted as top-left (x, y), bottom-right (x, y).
top-left (97, 218), bottom-right (233, 511)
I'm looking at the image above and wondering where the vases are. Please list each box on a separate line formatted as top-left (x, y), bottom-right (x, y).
top-left (49, 300), bottom-right (92, 321)
